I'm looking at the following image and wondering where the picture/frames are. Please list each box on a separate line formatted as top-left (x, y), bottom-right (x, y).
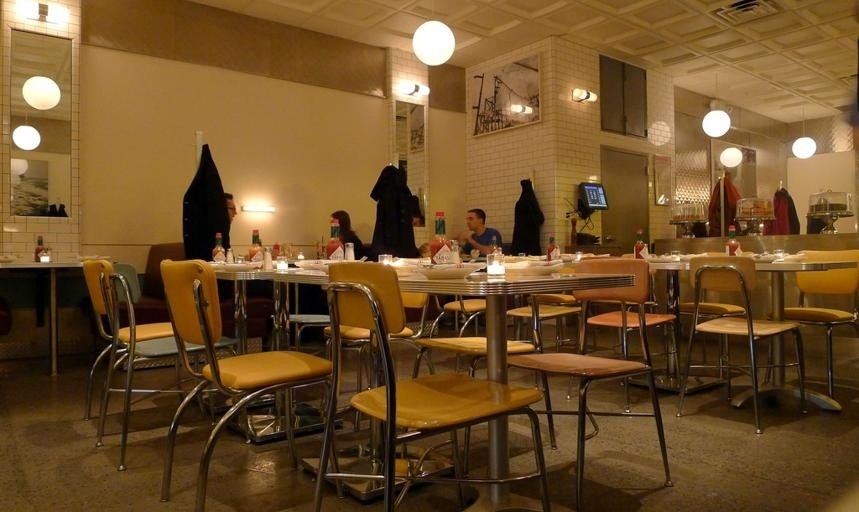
top-left (466, 48), bottom-right (542, 139)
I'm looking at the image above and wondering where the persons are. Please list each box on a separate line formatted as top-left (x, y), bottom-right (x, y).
top-left (329, 209), bottom-right (367, 260)
top-left (408, 193), bottom-right (430, 258)
top-left (459, 208), bottom-right (504, 258)
top-left (224, 192), bottom-right (238, 225)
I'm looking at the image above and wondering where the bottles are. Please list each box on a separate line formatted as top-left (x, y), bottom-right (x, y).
top-left (429, 208), bottom-right (460, 262)
top-left (465, 235), bottom-right (610, 259)
top-left (210, 218), bottom-right (422, 277)
top-left (726, 222), bottom-right (740, 259)
top-left (35, 234), bottom-right (47, 262)
top-left (570, 217), bottom-right (577, 245)
top-left (633, 226), bottom-right (646, 259)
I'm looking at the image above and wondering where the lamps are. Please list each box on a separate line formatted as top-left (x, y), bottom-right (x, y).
top-left (717, 147), bottom-right (742, 169)
top-left (16, 0), bottom-right (74, 26)
top-left (509, 103), bottom-right (534, 116)
top-left (698, 63), bottom-right (734, 139)
top-left (569, 82), bottom-right (601, 108)
top-left (792, 101), bottom-right (819, 160)
top-left (395, 78), bottom-right (432, 100)
top-left (11, 111), bottom-right (43, 151)
top-left (20, 73), bottom-right (62, 113)
top-left (411, 18), bottom-right (456, 69)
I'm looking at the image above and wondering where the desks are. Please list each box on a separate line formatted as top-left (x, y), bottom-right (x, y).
top-left (1, 256), bottom-right (91, 379)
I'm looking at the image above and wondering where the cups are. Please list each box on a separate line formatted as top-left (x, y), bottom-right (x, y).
top-left (488, 253), bottom-right (504, 275)
top-left (671, 251), bottom-right (681, 262)
top-left (40, 254), bottom-right (50, 263)
top-left (775, 250), bottom-right (784, 261)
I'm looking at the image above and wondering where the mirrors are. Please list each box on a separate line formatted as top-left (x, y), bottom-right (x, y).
top-left (394, 99), bottom-right (427, 228)
top-left (8, 24), bottom-right (73, 218)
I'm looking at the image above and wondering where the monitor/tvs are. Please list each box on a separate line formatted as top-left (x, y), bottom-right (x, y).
top-left (579, 182), bottom-right (608, 210)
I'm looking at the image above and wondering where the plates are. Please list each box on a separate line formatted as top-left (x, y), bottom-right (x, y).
top-left (752, 257), bottom-right (777, 263)
top-left (506, 261), bottom-right (564, 276)
top-left (649, 258), bottom-right (671, 262)
top-left (1, 255), bottom-right (18, 263)
top-left (414, 262), bottom-right (483, 279)
